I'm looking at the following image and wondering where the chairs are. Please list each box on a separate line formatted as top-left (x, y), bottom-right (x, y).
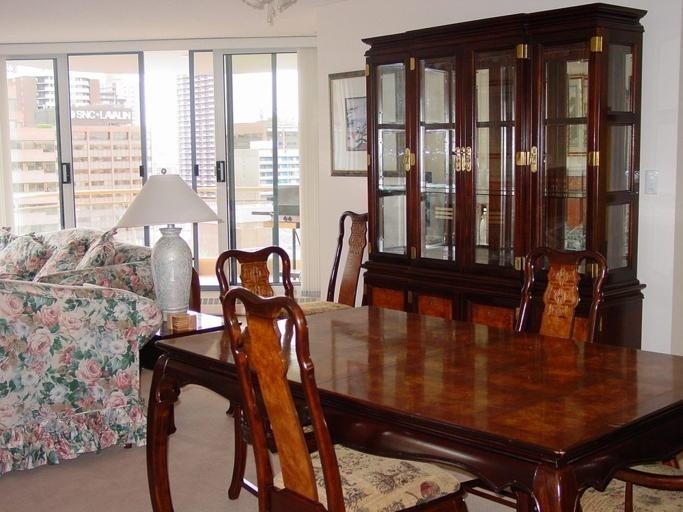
top-left (223, 286), bottom-right (480, 512)
top-left (214, 244), bottom-right (320, 501)
top-left (572, 456), bottom-right (683, 512)
top-left (511, 248), bottom-right (606, 341)
top-left (325, 211), bottom-right (371, 308)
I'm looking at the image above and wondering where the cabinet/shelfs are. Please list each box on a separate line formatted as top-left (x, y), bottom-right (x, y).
top-left (361, 3), bottom-right (648, 350)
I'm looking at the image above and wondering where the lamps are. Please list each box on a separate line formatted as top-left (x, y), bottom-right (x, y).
top-left (113, 174), bottom-right (225, 315)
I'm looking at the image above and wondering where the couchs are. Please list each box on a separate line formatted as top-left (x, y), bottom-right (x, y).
top-left (1, 228), bottom-right (165, 475)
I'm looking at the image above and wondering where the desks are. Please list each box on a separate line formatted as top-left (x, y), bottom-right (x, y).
top-left (146, 306), bottom-right (682, 510)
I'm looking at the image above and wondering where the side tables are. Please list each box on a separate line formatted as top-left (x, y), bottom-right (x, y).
top-left (139, 309), bottom-right (242, 435)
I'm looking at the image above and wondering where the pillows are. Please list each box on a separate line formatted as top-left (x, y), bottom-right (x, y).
top-left (1, 227), bottom-right (118, 280)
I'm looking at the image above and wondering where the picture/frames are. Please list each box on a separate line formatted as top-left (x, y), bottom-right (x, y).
top-left (329, 69), bottom-right (402, 177)
